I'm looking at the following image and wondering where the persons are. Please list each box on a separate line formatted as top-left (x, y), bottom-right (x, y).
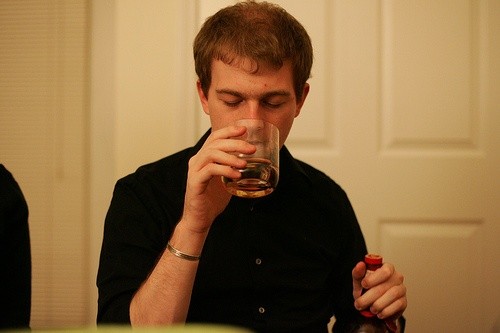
top-left (0, 164), bottom-right (32, 333)
top-left (96, 0), bottom-right (408, 333)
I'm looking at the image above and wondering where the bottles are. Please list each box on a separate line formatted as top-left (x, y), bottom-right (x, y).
top-left (344, 253), bottom-right (391, 333)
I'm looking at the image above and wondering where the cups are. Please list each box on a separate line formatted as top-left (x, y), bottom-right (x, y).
top-left (221, 119), bottom-right (280, 198)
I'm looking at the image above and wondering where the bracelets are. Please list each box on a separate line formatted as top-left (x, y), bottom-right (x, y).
top-left (166, 243), bottom-right (200, 261)
